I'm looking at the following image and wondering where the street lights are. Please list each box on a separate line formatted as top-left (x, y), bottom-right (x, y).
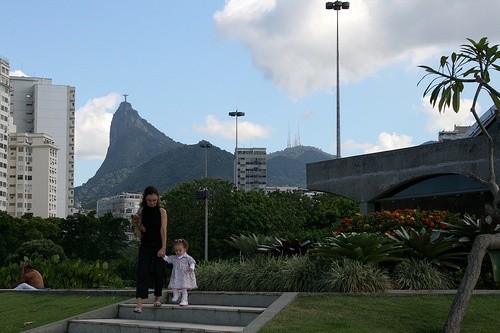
top-left (229, 109), bottom-right (246, 186)
top-left (200, 142), bottom-right (212, 261)
top-left (326, 1), bottom-right (350, 156)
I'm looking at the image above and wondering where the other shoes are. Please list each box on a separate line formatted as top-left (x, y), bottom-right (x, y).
top-left (154, 302), bottom-right (162, 307)
top-left (179, 300), bottom-right (189, 306)
top-left (172, 292), bottom-right (181, 302)
top-left (133, 309), bottom-right (143, 313)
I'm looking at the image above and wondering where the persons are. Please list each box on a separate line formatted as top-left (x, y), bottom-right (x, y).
top-left (161, 238), bottom-right (198, 306)
top-left (14, 264), bottom-right (43, 289)
top-left (132, 187), bottom-right (167, 313)
